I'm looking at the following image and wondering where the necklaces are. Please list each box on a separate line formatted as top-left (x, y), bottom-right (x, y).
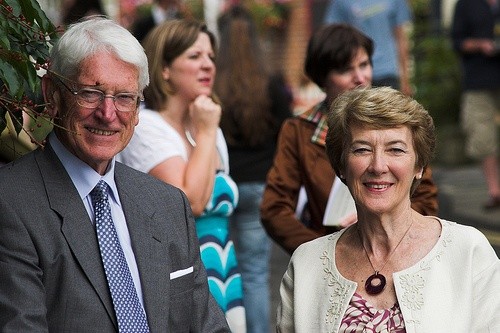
top-left (356, 220), bottom-right (413, 295)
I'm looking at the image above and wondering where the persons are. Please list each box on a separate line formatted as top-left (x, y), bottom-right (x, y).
top-left (276, 85), bottom-right (500, 333)
top-left (60, 0), bottom-right (293, 333)
top-left (116, 19), bottom-right (247, 333)
top-left (322, 0), bottom-right (413, 98)
top-left (449, 0), bottom-right (500, 211)
top-left (0, 17), bottom-right (231, 333)
top-left (259, 24), bottom-right (438, 256)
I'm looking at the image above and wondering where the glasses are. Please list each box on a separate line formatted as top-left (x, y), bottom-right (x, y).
top-left (58, 79), bottom-right (144, 112)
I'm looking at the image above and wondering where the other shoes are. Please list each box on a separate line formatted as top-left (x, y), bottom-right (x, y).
top-left (484, 196), bottom-right (500, 209)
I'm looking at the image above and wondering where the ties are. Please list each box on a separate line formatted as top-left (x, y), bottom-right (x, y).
top-left (89, 180), bottom-right (150, 333)
